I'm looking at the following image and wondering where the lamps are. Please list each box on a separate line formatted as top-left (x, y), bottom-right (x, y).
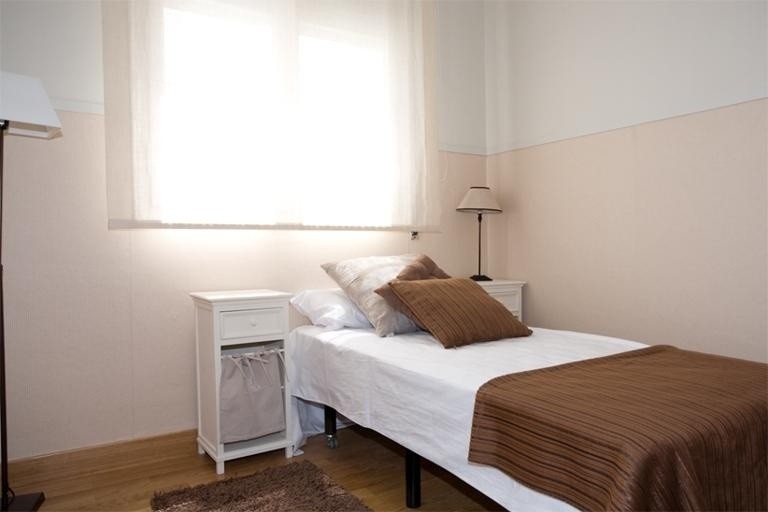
top-left (0, 70), bottom-right (62, 512)
top-left (456, 185), bottom-right (503, 280)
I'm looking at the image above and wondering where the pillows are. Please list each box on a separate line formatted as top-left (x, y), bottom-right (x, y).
top-left (291, 254), bottom-right (533, 350)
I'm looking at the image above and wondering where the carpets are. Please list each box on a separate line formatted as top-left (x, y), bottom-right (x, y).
top-left (151, 457), bottom-right (373, 512)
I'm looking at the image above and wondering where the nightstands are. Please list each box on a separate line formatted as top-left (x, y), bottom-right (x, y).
top-left (189, 289), bottom-right (296, 476)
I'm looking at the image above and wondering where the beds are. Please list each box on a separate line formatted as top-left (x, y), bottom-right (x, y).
top-left (292, 322), bottom-right (767, 512)
